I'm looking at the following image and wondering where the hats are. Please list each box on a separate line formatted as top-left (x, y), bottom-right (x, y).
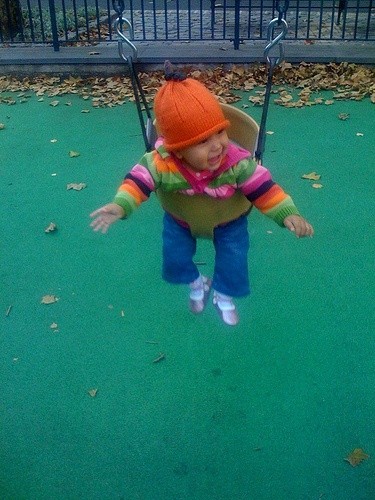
top-left (153, 60), bottom-right (231, 151)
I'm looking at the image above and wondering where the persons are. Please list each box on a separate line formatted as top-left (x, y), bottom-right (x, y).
top-left (89, 72), bottom-right (314, 325)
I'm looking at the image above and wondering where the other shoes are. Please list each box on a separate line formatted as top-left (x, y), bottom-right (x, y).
top-left (213, 290), bottom-right (239, 326)
top-left (188, 278), bottom-right (210, 314)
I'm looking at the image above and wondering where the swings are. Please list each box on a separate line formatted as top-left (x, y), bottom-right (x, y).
top-left (112, 0), bottom-right (290, 242)
